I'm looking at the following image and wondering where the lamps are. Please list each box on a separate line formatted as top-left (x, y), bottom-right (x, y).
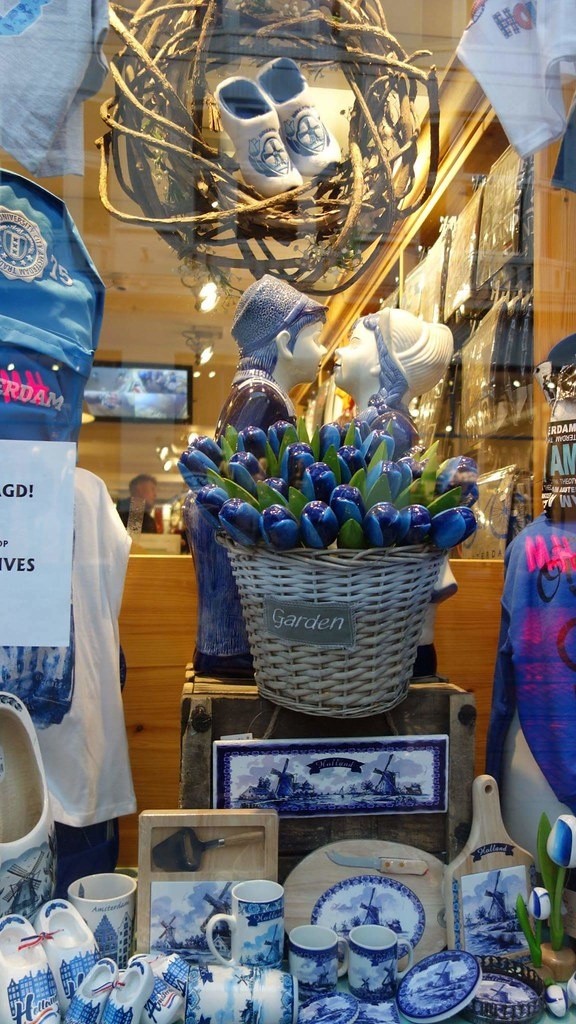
top-left (180, 256), bottom-right (220, 313)
top-left (180, 327), bottom-right (222, 366)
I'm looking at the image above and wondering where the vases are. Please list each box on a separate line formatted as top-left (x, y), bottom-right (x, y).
top-left (215, 531), bottom-right (450, 719)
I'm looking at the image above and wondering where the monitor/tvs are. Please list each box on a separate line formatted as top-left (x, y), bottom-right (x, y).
top-left (83, 359), bottom-right (194, 425)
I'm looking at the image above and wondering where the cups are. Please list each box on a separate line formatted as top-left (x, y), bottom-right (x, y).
top-left (206, 879), bottom-right (286, 970)
top-left (67, 872), bottom-right (138, 969)
top-left (185, 965), bottom-right (295, 1024)
top-left (288, 925), bottom-right (347, 1001)
top-left (347, 924), bottom-right (413, 1001)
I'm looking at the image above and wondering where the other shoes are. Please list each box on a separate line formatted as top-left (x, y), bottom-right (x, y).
top-left (213, 75), bottom-right (302, 196)
top-left (258, 57), bottom-right (340, 176)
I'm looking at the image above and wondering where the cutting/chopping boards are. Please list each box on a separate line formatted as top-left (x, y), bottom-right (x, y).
top-left (444, 774), bottom-right (540, 964)
top-left (284, 838), bottom-right (448, 976)
top-left (136, 809), bottom-right (279, 957)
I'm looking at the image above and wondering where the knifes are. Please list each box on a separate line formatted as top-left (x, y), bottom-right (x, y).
top-left (325, 850), bottom-right (428, 876)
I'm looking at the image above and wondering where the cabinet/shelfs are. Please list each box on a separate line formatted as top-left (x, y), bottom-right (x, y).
top-left (289, 56), bottom-right (576, 522)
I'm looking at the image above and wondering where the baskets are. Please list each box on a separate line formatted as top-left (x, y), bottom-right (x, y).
top-left (211, 524), bottom-right (446, 718)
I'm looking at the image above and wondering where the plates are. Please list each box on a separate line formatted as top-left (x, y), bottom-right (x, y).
top-left (396, 950), bottom-right (482, 1022)
top-left (297, 992), bottom-right (400, 1024)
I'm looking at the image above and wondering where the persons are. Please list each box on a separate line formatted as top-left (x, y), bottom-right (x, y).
top-left (116, 474), bottom-right (159, 535)
top-left (185, 275), bottom-right (455, 679)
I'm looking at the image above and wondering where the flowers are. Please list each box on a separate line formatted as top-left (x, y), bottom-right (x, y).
top-left (535, 812), bottom-right (576, 951)
top-left (178, 406), bottom-right (479, 549)
top-left (516, 887), bottom-right (551, 968)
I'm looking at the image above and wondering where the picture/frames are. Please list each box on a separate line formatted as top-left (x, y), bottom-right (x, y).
top-left (213, 734), bottom-right (449, 818)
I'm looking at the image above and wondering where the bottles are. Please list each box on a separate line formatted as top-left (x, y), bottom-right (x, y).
top-left (147, 484), bottom-right (186, 534)
